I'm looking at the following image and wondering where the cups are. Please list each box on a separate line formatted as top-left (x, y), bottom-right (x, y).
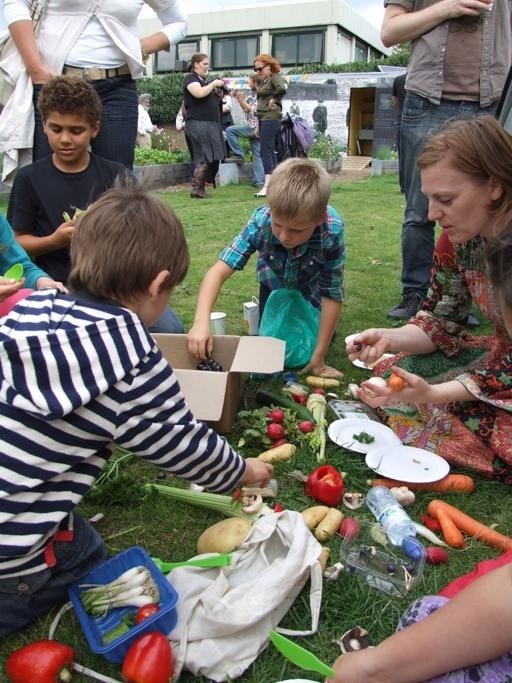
top-left (210, 311), bottom-right (226, 336)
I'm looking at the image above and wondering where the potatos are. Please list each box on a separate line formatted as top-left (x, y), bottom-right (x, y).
top-left (306, 376), bottom-right (340, 388)
top-left (258, 443), bottom-right (296, 464)
top-left (197, 517), bottom-right (252, 553)
top-left (300, 505), bottom-right (345, 573)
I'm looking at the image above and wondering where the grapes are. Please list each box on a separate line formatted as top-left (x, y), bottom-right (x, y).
top-left (197, 352), bottom-right (222, 371)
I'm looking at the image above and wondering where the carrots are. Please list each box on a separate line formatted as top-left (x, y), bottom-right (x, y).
top-left (371, 474), bottom-right (512, 552)
top-left (388, 373), bottom-right (406, 392)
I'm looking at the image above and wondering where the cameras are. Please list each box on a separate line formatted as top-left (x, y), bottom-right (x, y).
top-left (216, 78), bottom-right (231, 95)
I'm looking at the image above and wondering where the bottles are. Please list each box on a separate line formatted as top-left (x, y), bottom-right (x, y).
top-left (365, 482), bottom-right (426, 561)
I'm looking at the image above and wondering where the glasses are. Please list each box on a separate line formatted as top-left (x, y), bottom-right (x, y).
top-left (253, 65), bottom-right (268, 73)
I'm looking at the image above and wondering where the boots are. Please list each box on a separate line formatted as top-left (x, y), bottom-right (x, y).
top-left (191, 163), bottom-right (213, 198)
top-left (253, 174), bottom-right (272, 197)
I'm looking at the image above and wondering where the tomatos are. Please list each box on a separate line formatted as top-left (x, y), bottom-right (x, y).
top-left (304, 465), bottom-right (345, 505)
top-left (5, 639), bottom-right (75, 683)
top-left (121, 632), bottom-right (175, 683)
top-left (136, 604), bottom-right (160, 624)
top-left (341, 517), bottom-right (360, 539)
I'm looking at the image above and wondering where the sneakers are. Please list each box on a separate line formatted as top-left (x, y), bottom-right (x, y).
top-left (464, 314), bottom-right (480, 328)
top-left (387, 292), bottom-right (429, 320)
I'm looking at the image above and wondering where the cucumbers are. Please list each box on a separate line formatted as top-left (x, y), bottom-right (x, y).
top-left (256, 390), bottom-right (317, 425)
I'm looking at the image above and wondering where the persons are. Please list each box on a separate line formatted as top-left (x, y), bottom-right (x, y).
top-left (132, 47), bottom-right (316, 200)
top-left (343, 110), bottom-right (512, 419)
top-left (0, 0), bottom-right (191, 190)
top-left (0, 162), bottom-right (275, 642)
top-left (379, 2), bottom-right (512, 322)
top-left (184, 155), bottom-right (349, 380)
top-left (324, 559), bottom-right (512, 682)
top-left (0, 205), bottom-right (71, 317)
top-left (7, 71), bottom-right (189, 334)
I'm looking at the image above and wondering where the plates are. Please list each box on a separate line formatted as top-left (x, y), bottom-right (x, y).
top-left (365, 446), bottom-right (450, 483)
top-left (328, 416), bottom-right (395, 451)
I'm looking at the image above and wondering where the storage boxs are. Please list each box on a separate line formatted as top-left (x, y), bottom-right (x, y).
top-left (146, 330), bottom-right (291, 439)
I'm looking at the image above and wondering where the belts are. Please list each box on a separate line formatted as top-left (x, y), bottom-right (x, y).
top-left (63, 63), bottom-right (132, 84)
